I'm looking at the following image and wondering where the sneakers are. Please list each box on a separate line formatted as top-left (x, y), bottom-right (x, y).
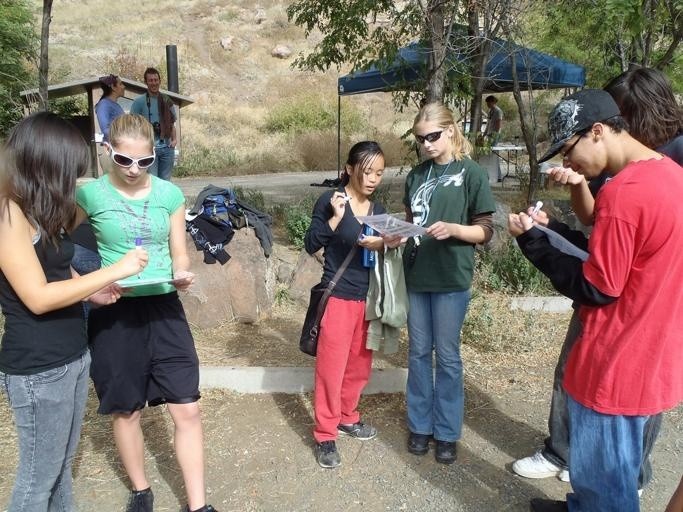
top-left (185, 503), bottom-right (216, 512)
top-left (511, 450), bottom-right (570, 483)
top-left (409, 430), bottom-right (457, 464)
top-left (339, 422), bottom-right (378, 442)
top-left (126, 489), bottom-right (155, 512)
top-left (315, 436), bottom-right (342, 470)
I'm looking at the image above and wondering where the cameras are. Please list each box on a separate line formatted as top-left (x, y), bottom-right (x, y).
top-left (150, 121), bottom-right (161, 137)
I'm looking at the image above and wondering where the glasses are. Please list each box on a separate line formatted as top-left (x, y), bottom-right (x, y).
top-left (560, 132), bottom-right (587, 160)
top-left (415, 128), bottom-right (445, 144)
top-left (110, 146), bottom-right (156, 169)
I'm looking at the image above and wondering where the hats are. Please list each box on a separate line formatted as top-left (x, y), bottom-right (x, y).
top-left (536, 89), bottom-right (620, 165)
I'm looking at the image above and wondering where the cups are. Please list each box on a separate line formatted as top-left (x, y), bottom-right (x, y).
top-left (515, 136), bottom-right (520, 146)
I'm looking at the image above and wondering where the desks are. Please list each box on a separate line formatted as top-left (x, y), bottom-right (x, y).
top-left (477, 145), bottom-right (526, 190)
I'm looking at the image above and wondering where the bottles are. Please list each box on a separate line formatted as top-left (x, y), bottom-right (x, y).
top-left (362, 224), bottom-right (376, 267)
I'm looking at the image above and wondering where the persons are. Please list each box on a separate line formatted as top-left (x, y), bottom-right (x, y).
top-left (67, 114), bottom-right (217, 512)
top-left (92, 73), bottom-right (129, 176)
top-left (1, 113), bottom-right (149, 512)
top-left (130, 67), bottom-right (177, 182)
top-left (381, 101), bottom-right (496, 465)
top-left (481, 96), bottom-right (503, 182)
top-left (297, 142), bottom-right (399, 468)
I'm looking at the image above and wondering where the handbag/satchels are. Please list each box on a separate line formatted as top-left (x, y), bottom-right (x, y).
top-left (299, 282), bottom-right (332, 356)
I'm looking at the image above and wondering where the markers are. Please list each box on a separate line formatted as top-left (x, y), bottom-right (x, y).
top-left (136, 238), bottom-right (142, 278)
top-left (337, 196), bottom-right (352, 201)
top-left (528, 200), bottom-right (544, 223)
